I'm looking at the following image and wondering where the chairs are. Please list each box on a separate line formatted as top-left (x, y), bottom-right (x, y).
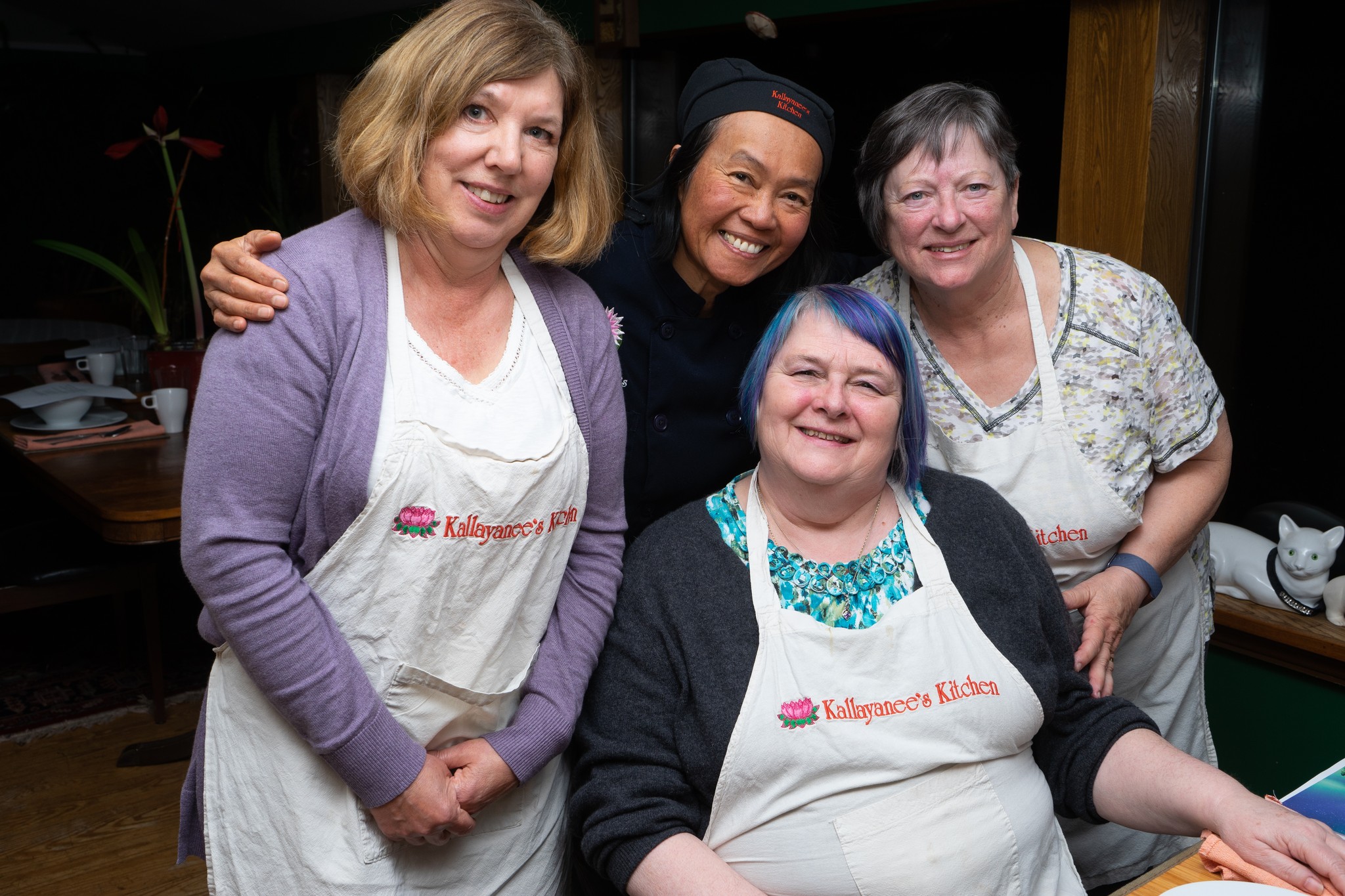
top-left (29, 283), bottom-right (141, 336)
top-left (0, 446), bottom-right (173, 725)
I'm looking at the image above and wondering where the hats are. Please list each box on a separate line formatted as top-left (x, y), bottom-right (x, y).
top-left (674, 57), bottom-right (838, 167)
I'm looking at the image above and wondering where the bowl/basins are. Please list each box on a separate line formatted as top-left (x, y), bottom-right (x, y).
top-left (33, 396), bottom-right (94, 426)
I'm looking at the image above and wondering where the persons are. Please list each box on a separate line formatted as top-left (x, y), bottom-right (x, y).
top-left (562, 285), bottom-right (1345, 896)
top-left (853, 83), bottom-right (1232, 896)
top-left (176, 20), bottom-right (629, 896)
top-left (200, 58), bottom-right (837, 547)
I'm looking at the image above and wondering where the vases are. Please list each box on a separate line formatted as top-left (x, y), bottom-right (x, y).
top-left (145, 342), bottom-right (205, 416)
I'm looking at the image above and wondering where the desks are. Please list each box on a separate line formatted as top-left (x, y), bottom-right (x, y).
top-left (0, 370), bottom-right (191, 720)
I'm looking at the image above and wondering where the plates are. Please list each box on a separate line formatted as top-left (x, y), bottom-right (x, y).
top-left (9, 409), bottom-right (128, 431)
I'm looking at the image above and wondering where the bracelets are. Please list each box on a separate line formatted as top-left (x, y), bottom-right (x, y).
top-left (1107, 552), bottom-right (1163, 608)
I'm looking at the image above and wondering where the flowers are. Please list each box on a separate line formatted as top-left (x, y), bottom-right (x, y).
top-left (21, 105), bottom-right (225, 353)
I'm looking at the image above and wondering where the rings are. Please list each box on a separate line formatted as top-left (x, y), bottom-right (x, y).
top-left (1109, 657), bottom-right (1114, 662)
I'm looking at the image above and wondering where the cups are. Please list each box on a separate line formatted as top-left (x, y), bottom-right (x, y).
top-left (141, 387), bottom-right (188, 434)
top-left (76, 353), bottom-right (116, 386)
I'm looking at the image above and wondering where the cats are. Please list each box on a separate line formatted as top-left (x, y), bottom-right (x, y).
top-left (1206, 512), bottom-right (1345, 618)
